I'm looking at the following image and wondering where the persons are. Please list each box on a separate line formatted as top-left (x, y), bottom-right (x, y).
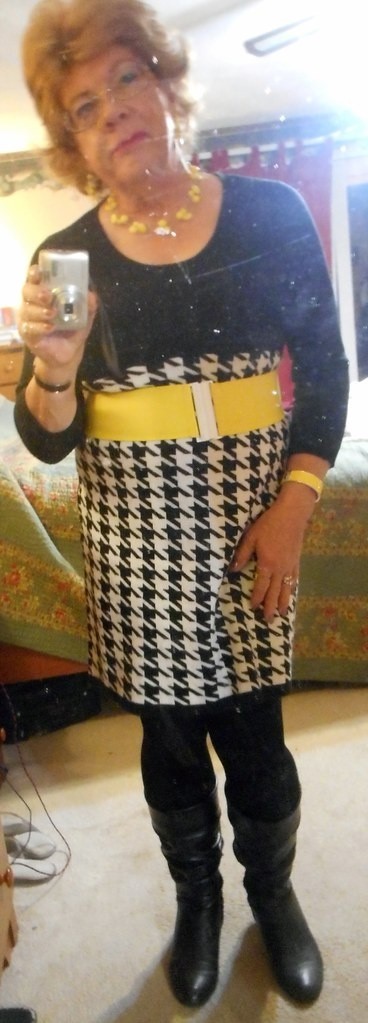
top-left (14, 0), bottom-right (350, 1007)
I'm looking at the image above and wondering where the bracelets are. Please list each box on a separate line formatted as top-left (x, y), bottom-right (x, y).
top-left (278, 470), bottom-right (325, 501)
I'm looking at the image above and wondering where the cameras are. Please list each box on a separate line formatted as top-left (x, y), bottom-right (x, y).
top-left (38, 248), bottom-right (90, 331)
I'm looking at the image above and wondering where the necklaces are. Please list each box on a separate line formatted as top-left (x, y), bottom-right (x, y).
top-left (106, 163), bottom-right (204, 238)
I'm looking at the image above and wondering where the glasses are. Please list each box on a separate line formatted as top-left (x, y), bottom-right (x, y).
top-left (56, 55), bottom-right (164, 134)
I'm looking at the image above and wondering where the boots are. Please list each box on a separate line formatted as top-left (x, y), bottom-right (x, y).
top-left (225, 793), bottom-right (330, 1004)
top-left (145, 783), bottom-right (226, 1008)
